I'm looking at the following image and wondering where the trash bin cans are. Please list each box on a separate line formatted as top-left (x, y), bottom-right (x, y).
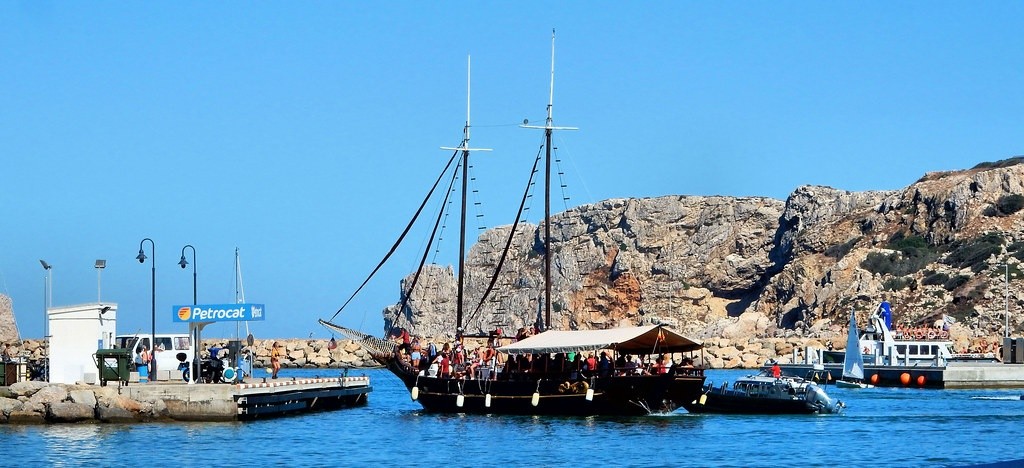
top-left (135, 363), bottom-right (148, 383)
top-left (96, 349), bottom-right (132, 386)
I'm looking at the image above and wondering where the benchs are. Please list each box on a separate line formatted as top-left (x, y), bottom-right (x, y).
top-left (474, 359), bottom-right (493, 379)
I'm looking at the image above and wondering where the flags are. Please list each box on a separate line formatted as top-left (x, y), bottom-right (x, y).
top-left (946, 316), bottom-right (954, 325)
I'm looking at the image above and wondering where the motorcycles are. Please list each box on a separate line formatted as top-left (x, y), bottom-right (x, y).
top-left (176, 353), bottom-right (231, 384)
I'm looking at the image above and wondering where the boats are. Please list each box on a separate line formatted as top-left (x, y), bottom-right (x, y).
top-left (701, 358), bottom-right (848, 416)
top-left (759, 316), bottom-right (957, 390)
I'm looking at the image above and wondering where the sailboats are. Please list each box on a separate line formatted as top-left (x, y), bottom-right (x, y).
top-left (835, 307), bottom-right (875, 389)
top-left (317, 25), bottom-right (710, 419)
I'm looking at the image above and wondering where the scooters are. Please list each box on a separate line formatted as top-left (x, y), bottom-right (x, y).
top-left (26, 359), bottom-right (49, 382)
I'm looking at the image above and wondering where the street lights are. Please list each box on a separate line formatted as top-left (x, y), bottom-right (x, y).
top-left (135, 238), bottom-right (157, 382)
top-left (178, 244), bottom-right (198, 382)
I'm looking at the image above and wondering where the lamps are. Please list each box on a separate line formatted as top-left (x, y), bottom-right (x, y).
top-left (100, 307), bottom-right (112, 314)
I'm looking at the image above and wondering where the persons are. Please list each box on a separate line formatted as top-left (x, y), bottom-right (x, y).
top-left (962, 343), bottom-right (998, 353)
top-left (271, 341), bottom-right (281, 378)
top-left (863, 346), bottom-right (870, 354)
top-left (396, 327), bottom-right (534, 380)
top-left (141, 344), bottom-right (151, 380)
top-left (768, 362), bottom-right (781, 380)
top-left (949, 346), bottom-right (958, 354)
top-left (898, 320), bottom-right (947, 339)
top-left (530, 327), bottom-right (694, 376)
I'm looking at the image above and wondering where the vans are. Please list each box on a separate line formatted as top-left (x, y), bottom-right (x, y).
top-left (114, 334), bottom-right (195, 374)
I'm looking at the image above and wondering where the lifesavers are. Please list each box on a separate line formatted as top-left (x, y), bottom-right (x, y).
top-left (980, 339), bottom-right (987, 347)
top-left (903, 331), bottom-right (911, 339)
top-left (993, 341), bottom-right (998, 349)
top-left (927, 330), bottom-right (936, 339)
top-left (916, 331), bottom-right (923, 339)
top-left (940, 331), bottom-right (948, 339)
